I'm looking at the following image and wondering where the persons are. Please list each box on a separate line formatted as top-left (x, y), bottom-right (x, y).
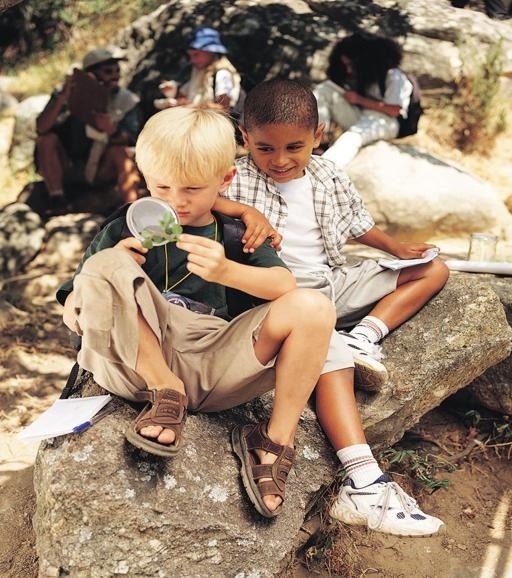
top-left (57, 101), bottom-right (338, 524)
top-left (32, 47), bottom-right (145, 212)
top-left (153, 26), bottom-right (250, 159)
top-left (208, 77), bottom-right (453, 539)
top-left (312, 29), bottom-right (424, 172)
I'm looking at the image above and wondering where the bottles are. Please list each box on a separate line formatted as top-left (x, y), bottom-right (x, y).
top-left (467, 232), bottom-right (498, 262)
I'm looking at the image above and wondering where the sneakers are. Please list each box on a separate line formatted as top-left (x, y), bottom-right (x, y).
top-left (328, 473), bottom-right (447, 539)
top-left (337, 328), bottom-right (388, 393)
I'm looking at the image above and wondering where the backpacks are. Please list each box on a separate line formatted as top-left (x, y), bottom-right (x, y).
top-left (212, 65), bottom-right (247, 123)
top-left (377, 67), bottom-right (425, 141)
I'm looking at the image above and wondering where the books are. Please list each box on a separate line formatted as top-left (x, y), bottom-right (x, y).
top-left (17, 393), bottom-right (114, 442)
top-left (445, 256), bottom-right (512, 276)
top-left (377, 243), bottom-right (441, 273)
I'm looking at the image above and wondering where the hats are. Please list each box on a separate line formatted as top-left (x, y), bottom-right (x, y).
top-left (82, 47), bottom-right (128, 69)
top-left (185, 26), bottom-right (228, 54)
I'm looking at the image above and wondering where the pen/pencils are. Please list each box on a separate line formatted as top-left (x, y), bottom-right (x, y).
top-left (71, 405), bottom-right (115, 433)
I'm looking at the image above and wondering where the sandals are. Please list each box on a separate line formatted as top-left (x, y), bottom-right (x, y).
top-left (125, 388), bottom-right (190, 457)
top-left (231, 421), bottom-right (299, 518)
